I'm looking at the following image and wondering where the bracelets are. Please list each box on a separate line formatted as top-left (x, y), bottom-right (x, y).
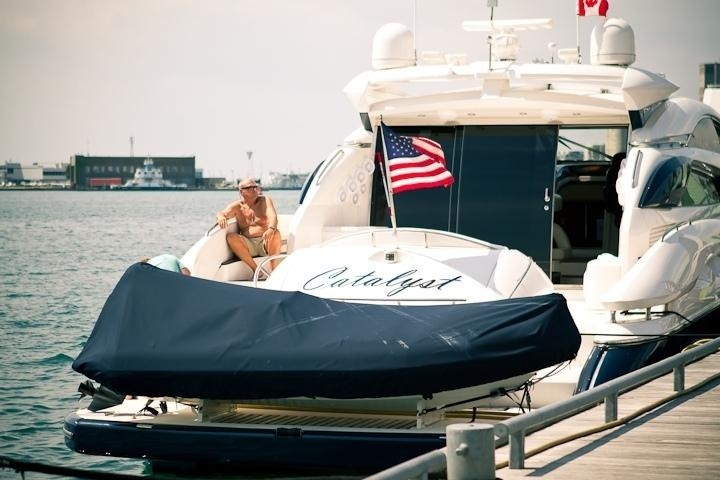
top-left (269, 226), bottom-right (278, 233)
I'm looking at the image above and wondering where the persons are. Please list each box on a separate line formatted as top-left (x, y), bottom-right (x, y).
top-left (141, 254), bottom-right (191, 275)
top-left (214, 177), bottom-right (281, 283)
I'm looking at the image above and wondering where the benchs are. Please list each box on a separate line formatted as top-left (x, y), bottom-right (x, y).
top-left (214, 252), bottom-right (287, 281)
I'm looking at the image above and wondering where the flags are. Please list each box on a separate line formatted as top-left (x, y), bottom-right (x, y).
top-left (381, 120), bottom-right (455, 195)
top-left (576, 1), bottom-right (610, 18)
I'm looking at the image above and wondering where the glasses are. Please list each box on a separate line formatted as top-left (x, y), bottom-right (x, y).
top-left (241, 185), bottom-right (258, 191)
